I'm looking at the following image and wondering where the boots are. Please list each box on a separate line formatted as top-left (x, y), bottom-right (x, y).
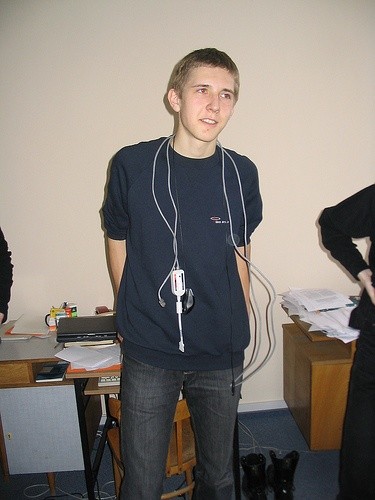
top-left (242, 454), bottom-right (266, 500)
top-left (268, 450), bottom-right (299, 500)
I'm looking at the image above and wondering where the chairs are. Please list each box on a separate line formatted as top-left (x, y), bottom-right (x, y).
top-left (107, 396), bottom-right (197, 500)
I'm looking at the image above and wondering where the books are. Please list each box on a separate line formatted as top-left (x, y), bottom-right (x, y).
top-left (34, 361), bottom-right (69, 383)
top-left (63, 337), bottom-right (116, 348)
top-left (0, 313), bottom-right (51, 341)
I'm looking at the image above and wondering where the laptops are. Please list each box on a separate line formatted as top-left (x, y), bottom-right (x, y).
top-left (56, 316), bottom-right (118, 342)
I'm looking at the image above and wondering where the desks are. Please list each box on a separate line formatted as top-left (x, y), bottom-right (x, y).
top-left (66, 364), bottom-right (184, 500)
top-left (280, 303), bottom-right (356, 450)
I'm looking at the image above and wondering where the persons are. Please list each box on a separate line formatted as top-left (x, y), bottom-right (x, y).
top-left (318, 183), bottom-right (375, 500)
top-left (102, 48), bottom-right (263, 500)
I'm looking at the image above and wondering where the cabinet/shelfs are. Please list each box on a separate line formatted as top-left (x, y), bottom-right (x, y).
top-left (0, 331), bottom-right (103, 498)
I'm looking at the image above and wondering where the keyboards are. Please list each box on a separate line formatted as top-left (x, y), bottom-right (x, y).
top-left (98, 376), bottom-right (121, 387)
